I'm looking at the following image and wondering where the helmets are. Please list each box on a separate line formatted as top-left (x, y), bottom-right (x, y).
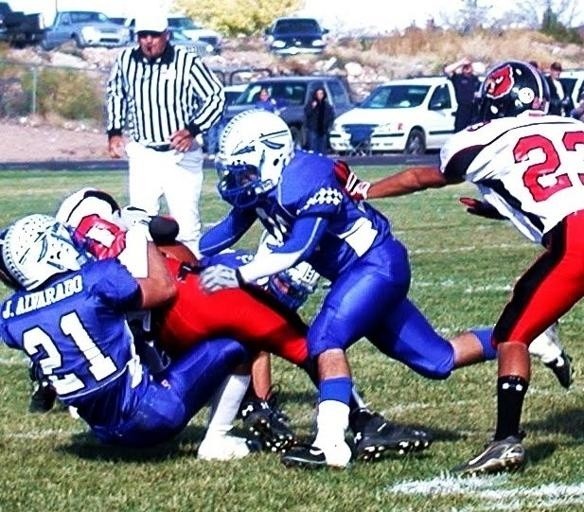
top-left (1, 214), bottom-right (81, 292)
top-left (1, 228), bottom-right (23, 289)
top-left (266, 261), bottom-right (322, 310)
top-left (54, 186), bottom-right (122, 235)
top-left (480, 59), bottom-right (552, 122)
top-left (213, 107), bottom-right (295, 209)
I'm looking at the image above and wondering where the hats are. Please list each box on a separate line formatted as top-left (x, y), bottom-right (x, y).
top-left (552, 62), bottom-right (562, 71)
top-left (133, 12), bottom-right (170, 35)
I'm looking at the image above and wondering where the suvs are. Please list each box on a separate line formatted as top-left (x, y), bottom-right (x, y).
top-left (163, 15), bottom-right (223, 55)
top-left (224, 76), bottom-right (355, 146)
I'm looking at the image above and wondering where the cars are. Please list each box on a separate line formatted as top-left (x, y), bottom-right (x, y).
top-left (265, 17), bottom-right (330, 57)
top-left (327, 75), bottom-right (485, 155)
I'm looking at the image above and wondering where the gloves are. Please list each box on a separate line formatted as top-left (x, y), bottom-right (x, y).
top-left (198, 263), bottom-right (242, 293)
top-left (332, 157), bottom-right (371, 201)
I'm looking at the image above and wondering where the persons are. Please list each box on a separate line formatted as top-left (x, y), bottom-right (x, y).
top-left (182, 111), bottom-right (576, 471)
top-left (527, 58), bottom-right (538, 72)
top-left (0, 205), bottom-right (263, 462)
top-left (545, 63), bottom-right (571, 118)
top-left (304, 88), bottom-right (335, 158)
top-left (442, 58), bottom-right (483, 133)
top-left (102, 11), bottom-right (225, 263)
top-left (333, 61), bottom-right (583, 479)
top-left (255, 88), bottom-right (277, 124)
top-left (52, 187), bottom-right (430, 460)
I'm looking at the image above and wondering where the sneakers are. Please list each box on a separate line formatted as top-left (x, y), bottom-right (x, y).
top-left (239, 401), bottom-right (300, 455)
top-left (539, 320), bottom-right (577, 390)
top-left (351, 410), bottom-right (432, 461)
top-left (448, 433), bottom-right (527, 475)
top-left (281, 436), bottom-right (352, 469)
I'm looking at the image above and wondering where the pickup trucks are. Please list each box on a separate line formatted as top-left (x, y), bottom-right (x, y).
top-left (42, 10), bottom-right (130, 50)
